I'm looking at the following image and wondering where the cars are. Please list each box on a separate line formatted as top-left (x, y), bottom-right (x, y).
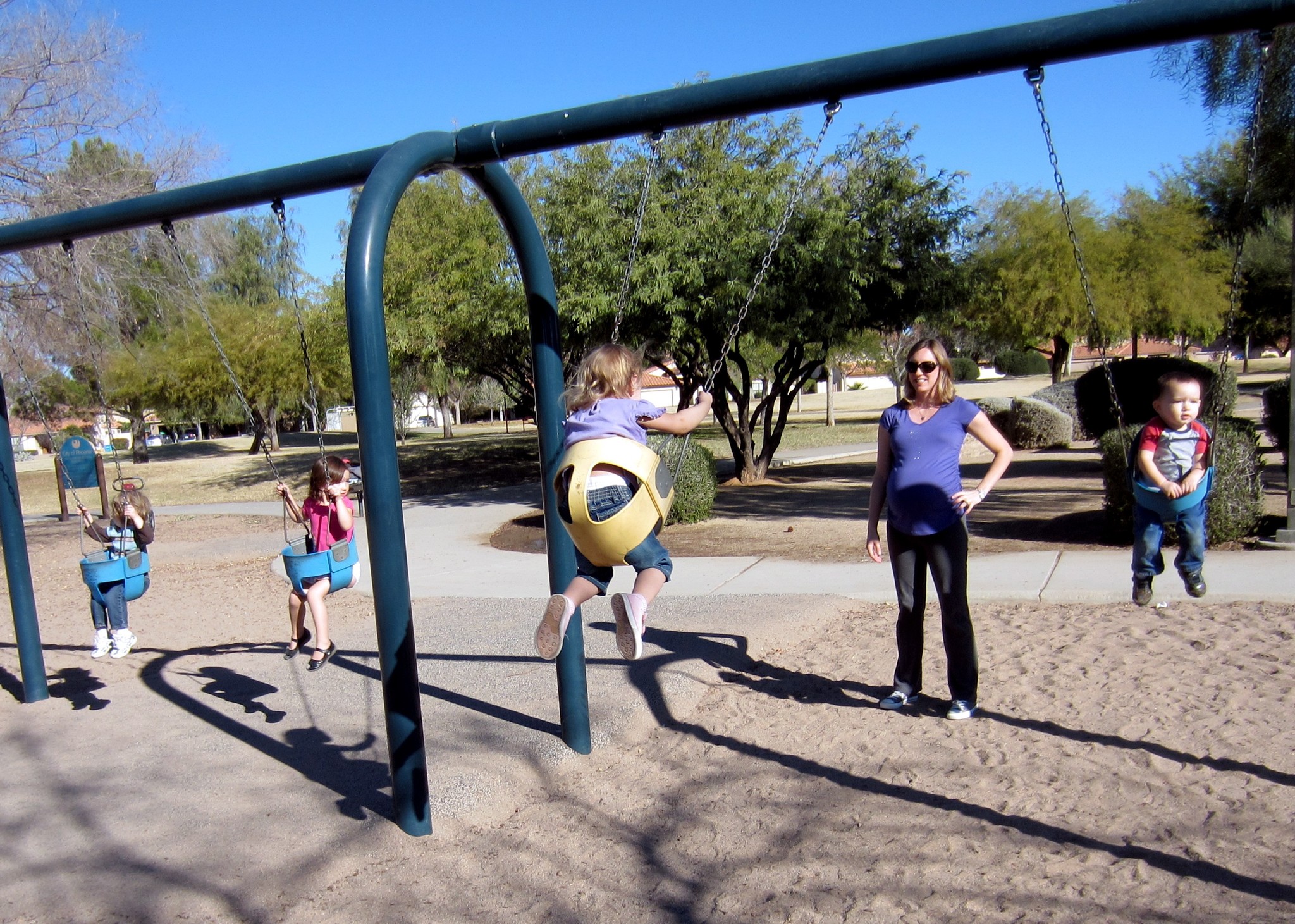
top-left (146, 430), bottom-right (196, 447)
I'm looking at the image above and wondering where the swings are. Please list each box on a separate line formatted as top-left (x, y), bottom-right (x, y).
top-left (1022, 25), bottom-right (1280, 525)
top-left (0, 243), bottom-right (151, 607)
top-left (552, 101), bottom-right (843, 566)
top-left (162, 201), bottom-right (359, 598)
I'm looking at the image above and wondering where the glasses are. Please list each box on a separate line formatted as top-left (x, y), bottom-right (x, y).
top-left (905, 361), bottom-right (940, 374)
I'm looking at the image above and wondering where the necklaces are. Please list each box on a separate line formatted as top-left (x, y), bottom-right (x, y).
top-left (915, 404), bottom-right (933, 421)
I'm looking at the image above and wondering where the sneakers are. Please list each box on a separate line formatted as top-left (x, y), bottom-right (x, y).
top-left (535, 594), bottom-right (575, 660)
top-left (611, 593), bottom-right (647, 661)
top-left (92, 632), bottom-right (114, 658)
top-left (110, 631), bottom-right (137, 658)
top-left (1133, 577), bottom-right (1152, 606)
top-left (946, 700), bottom-right (978, 719)
top-left (879, 691), bottom-right (918, 709)
top-left (1178, 570), bottom-right (1206, 597)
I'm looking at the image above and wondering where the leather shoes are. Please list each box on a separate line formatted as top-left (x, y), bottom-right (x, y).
top-left (284, 627), bottom-right (311, 660)
top-left (306, 638), bottom-right (337, 672)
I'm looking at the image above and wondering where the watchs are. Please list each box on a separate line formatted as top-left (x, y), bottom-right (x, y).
top-left (975, 487), bottom-right (986, 500)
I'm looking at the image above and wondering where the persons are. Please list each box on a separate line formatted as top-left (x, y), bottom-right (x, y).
top-left (275, 454), bottom-right (359, 672)
top-left (531, 343), bottom-right (713, 661)
top-left (86, 482), bottom-right (155, 660)
top-left (1127, 371), bottom-right (1215, 607)
top-left (866, 334), bottom-right (1016, 721)
